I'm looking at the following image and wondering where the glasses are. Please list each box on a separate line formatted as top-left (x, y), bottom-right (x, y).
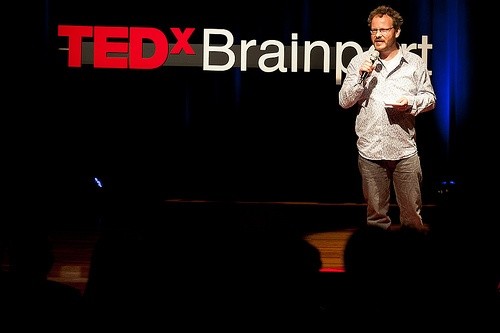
top-left (370, 27), bottom-right (394, 36)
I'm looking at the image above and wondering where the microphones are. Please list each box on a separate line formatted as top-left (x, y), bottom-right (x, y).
top-left (360, 51), bottom-right (381, 83)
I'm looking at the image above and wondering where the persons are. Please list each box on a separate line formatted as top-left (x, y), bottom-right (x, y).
top-left (338, 4), bottom-right (436, 229)
